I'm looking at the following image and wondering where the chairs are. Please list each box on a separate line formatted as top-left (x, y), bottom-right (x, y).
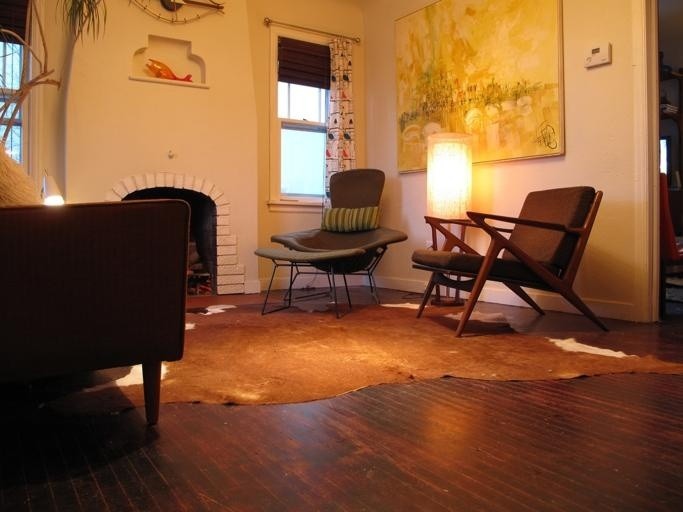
top-left (0, 199), bottom-right (191, 427)
top-left (410, 186), bottom-right (611, 339)
top-left (270, 168), bottom-right (408, 306)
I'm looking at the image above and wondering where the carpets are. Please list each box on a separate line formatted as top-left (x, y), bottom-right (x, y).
top-left (43, 301), bottom-right (683, 421)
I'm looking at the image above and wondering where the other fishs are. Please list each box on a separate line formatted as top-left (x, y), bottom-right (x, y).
top-left (145, 58), bottom-right (192, 83)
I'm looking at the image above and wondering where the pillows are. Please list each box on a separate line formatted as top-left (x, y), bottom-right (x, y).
top-left (320, 206), bottom-right (384, 232)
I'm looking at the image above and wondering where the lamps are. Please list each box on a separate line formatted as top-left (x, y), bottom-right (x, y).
top-left (39, 169), bottom-right (65, 205)
top-left (425, 132), bottom-right (474, 305)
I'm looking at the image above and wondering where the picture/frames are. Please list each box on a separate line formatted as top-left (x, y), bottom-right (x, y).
top-left (392, 0), bottom-right (565, 176)
